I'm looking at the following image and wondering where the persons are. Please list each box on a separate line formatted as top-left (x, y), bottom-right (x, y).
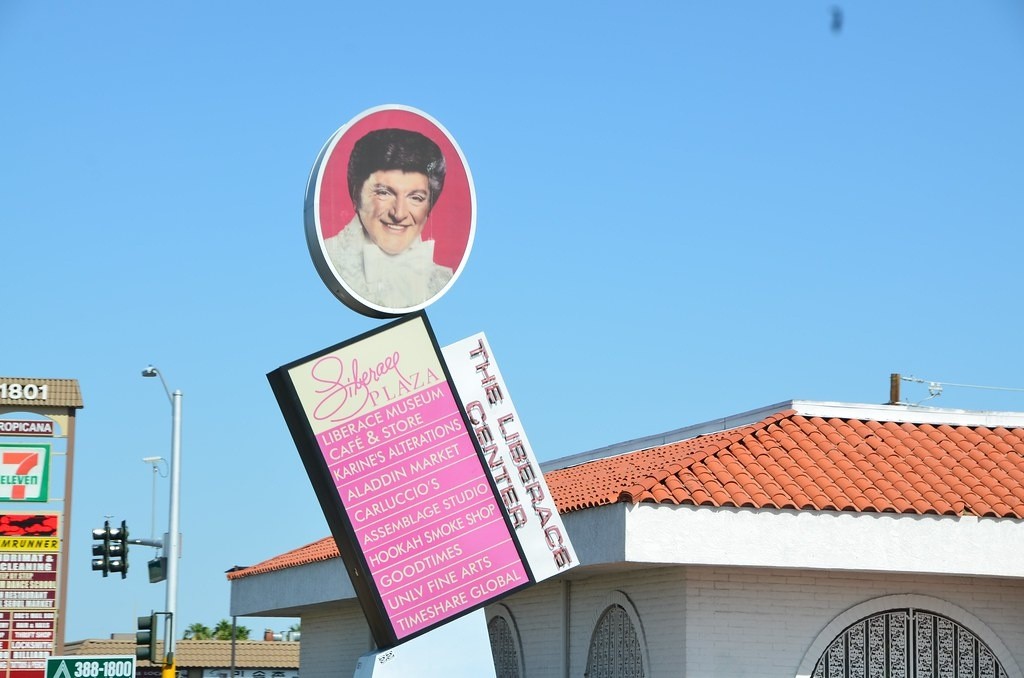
top-left (323, 128), bottom-right (453, 308)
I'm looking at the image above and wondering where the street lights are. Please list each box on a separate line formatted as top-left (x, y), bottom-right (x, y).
top-left (141, 366), bottom-right (183, 678)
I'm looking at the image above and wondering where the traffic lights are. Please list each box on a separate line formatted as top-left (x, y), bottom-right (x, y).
top-left (109, 520), bottom-right (129, 579)
top-left (136, 614), bottom-right (157, 662)
top-left (148, 557), bottom-right (167, 583)
top-left (92, 520), bottom-right (110, 577)
